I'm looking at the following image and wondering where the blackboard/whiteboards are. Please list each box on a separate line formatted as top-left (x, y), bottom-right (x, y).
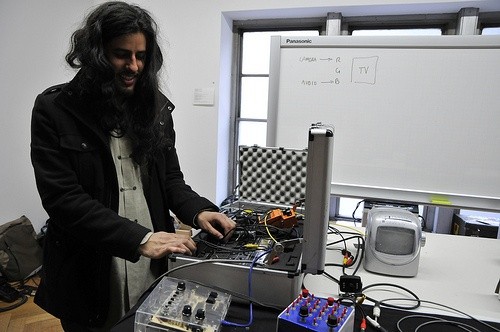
top-left (265, 36), bottom-right (500, 212)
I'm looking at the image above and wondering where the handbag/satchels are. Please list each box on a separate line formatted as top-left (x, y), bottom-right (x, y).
top-left (0, 215), bottom-right (44, 282)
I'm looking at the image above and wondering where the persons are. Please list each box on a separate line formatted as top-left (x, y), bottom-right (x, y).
top-left (31, 1), bottom-right (236, 332)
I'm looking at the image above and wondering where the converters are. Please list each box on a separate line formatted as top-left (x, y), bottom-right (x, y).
top-left (340, 275), bottom-right (362, 293)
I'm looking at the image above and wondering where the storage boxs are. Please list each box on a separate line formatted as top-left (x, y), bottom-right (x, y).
top-left (167, 122), bottom-right (335, 309)
top-left (451, 214), bottom-right (499, 238)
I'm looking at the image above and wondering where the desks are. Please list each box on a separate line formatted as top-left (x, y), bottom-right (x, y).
top-left (303, 221), bottom-right (499, 325)
